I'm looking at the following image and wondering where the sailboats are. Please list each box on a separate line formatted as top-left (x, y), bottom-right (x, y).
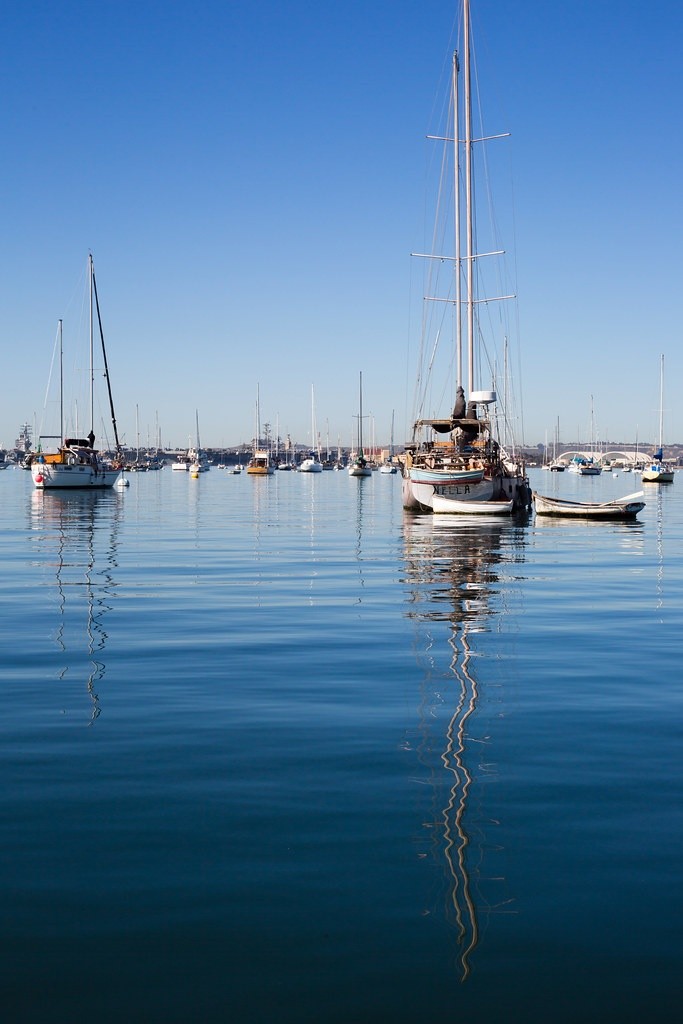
top-left (0, 2), bottom-right (674, 509)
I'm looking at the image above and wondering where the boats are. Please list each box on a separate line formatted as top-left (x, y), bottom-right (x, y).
top-left (432, 496), bottom-right (515, 513)
top-left (532, 492), bottom-right (646, 519)
